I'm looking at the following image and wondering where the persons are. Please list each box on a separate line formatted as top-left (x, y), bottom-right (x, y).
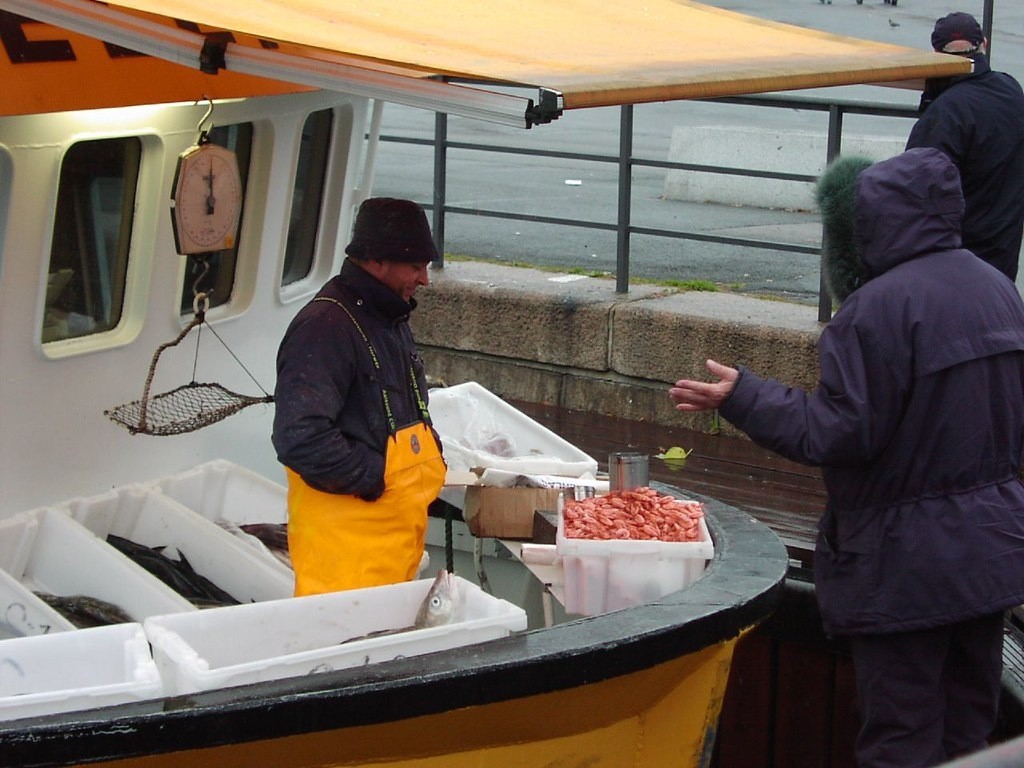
top-left (905, 12), bottom-right (1024, 290)
top-left (271, 196), bottom-right (447, 599)
top-left (668, 142), bottom-right (1024, 768)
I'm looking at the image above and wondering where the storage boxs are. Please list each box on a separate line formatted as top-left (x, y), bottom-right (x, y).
top-left (0, 382), bottom-right (713, 721)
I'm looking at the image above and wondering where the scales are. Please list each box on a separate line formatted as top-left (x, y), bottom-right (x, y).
top-left (101, 129), bottom-right (276, 438)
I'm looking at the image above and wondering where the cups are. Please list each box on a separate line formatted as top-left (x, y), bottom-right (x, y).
top-left (574, 485), bottom-right (595, 502)
top-left (608, 451), bottom-right (650, 493)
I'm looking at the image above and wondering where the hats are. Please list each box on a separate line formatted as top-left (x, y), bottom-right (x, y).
top-left (345, 198), bottom-right (439, 262)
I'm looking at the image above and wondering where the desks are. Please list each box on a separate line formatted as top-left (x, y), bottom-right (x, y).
top-left (436, 485), bottom-right (565, 628)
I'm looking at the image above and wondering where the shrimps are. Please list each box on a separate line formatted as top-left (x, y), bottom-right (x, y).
top-left (563, 485), bottom-right (705, 543)
